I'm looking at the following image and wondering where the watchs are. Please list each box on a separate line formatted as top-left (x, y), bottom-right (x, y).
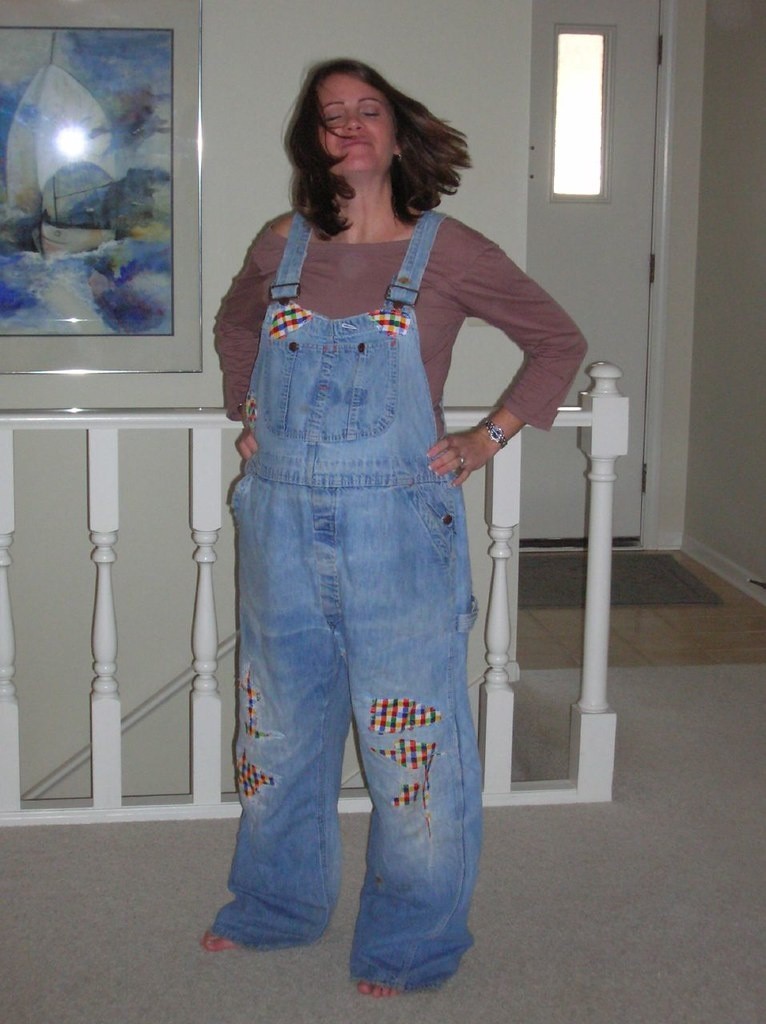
top-left (482, 418), bottom-right (508, 448)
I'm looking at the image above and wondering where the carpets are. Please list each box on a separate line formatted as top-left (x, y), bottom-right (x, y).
top-left (519, 552), bottom-right (722, 609)
top-left (0, 660), bottom-right (766, 1024)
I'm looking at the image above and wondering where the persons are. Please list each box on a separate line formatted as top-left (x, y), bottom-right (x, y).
top-left (204, 60), bottom-right (591, 996)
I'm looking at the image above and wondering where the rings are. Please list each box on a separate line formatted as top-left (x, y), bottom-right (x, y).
top-left (459, 456), bottom-right (464, 464)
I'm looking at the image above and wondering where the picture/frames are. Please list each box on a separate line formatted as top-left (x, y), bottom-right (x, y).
top-left (0, 1), bottom-right (203, 375)
